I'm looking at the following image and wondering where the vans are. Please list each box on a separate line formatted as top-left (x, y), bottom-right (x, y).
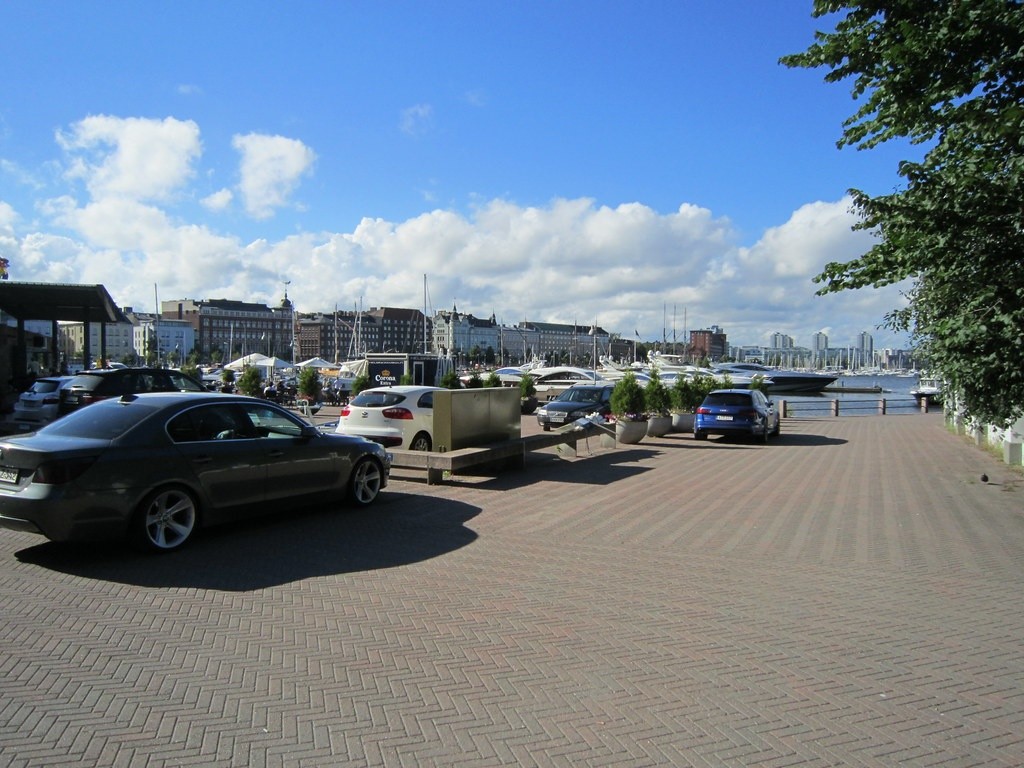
top-left (91, 362), bottom-right (127, 369)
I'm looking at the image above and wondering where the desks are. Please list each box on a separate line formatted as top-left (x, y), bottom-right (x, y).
top-left (330, 389), bottom-right (352, 403)
top-left (276, 390), bottom-right (289, 404)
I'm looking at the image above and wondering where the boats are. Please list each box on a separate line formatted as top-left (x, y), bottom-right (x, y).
top-left (456, 361), bottom-right (775, 399)
top-left (709, 363), bottom-right (838, 392)
top-left (910, 376), bottom-right (949, 405)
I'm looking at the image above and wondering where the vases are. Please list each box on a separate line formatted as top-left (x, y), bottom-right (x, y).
top-left (206, 384), bottom-right (216, 391)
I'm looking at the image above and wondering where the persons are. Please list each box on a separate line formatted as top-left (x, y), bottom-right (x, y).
top-left (215, 416), bottom-right (244, 439)
top-left (318, 375), bottom-right (348, 406)
top-left (262, 374), bottom-right (297, 403)
top-left (50, 361), bottom-right (70, 377)
top-left (199, 363), bottom-right (234, 393)
top-left (28, 357), bottom-right (39, 375)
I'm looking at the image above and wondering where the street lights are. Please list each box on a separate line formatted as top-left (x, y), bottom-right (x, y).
top-left (589, 323), bottom-right (598, 387)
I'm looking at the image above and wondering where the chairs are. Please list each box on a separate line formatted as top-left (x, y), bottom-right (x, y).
top-left (265, 390), bottom-right (277, 405)
top-left (321, 389), bottom-right (333, 407)
top-left (337, 390), bottom-right (350, 407)
top-left (282, 390), bottom-right (296, 407)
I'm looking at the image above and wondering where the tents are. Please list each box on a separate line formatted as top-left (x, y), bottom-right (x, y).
top-left (224, 353), bottom-right (365, 375)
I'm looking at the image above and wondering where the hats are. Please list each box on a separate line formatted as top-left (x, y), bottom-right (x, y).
top-left (268, 381), bottom-right (274, 385)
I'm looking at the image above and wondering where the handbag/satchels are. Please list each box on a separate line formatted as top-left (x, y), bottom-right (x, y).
top-left (336, 388), bottom-right (340, 398)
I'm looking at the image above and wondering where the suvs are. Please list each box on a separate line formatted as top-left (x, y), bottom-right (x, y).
top-left (13, 376), bottom-right (77, 432)
top-left (56, 367), bottom-right (212, 419)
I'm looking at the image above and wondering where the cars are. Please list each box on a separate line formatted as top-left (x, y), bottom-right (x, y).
top-left (693, 390), bottom-right (780, 443)
top-left (0, 391), bottom-right (390, 558)
top-left (537, 384), bottom-right (616, 431)
top-left (333, 386), bottom-right (449, 453)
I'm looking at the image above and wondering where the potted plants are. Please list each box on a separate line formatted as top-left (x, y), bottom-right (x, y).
top-left (150, 364), bottom-right (165, 387)
top-left (400, 368), bottom-right (413, 386)
top-left (181, 367), bottom-right (203, 392)
top-left (609, 367), bottom-right (648, 444)
top-left (297, 365), bottom-right (322, 416)
top-left (482, 368), bottom-right (503, 387)
top-left (465, 369), bottom-right (483, 388)
top-left (235, 364), bottom-right (266, 401)
top-left (671, 368), bottom-right (771, 430)
top-left (221, 369), bottom-right (236, 394)
top-left (349, 370), bottom-right (371, 403)
top-left (439, 369), bottom-right (462, 389)
top-left (516, 370), bottom-right (538, 415)
top-left (644, 363), bottom-right (672, 437)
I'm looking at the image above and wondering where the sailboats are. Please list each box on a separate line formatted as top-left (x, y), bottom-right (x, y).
top-left (336, 296), bottom-right (367, 392)
top-left (777, 346), bottom-right (921, 378)
